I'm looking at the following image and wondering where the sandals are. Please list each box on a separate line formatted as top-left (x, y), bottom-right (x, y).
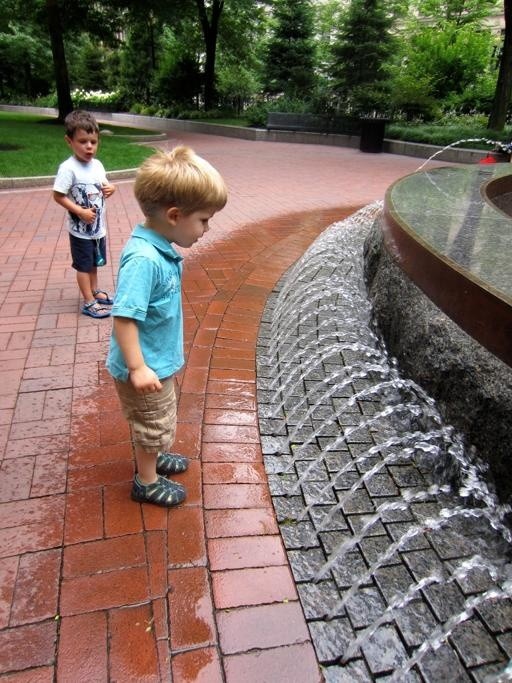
top-left (93, 289), bottom-right (113, 304)
top-left (131, 472), bottom-right (185, 505)
top-left (156, 452), bottom-right (188, 474)
top-left (81, 300), bottom-right (111, 318)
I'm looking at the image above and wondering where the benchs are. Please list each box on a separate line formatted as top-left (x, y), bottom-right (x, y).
top-left (267, 111), bottom-right (362, 136)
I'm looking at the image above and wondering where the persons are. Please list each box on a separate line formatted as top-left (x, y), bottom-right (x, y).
top-left (51, 109), bottom-right (115, 319)
top-left (104, 143), bottom-right (229, 506)
